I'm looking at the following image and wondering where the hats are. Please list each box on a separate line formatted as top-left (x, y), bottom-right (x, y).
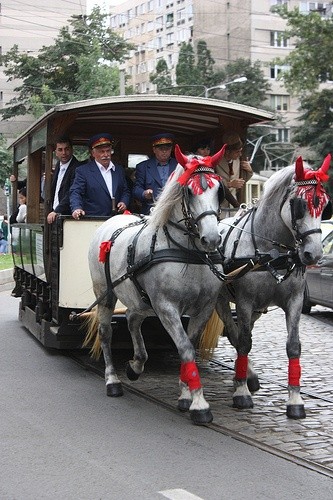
top-left (87, 134), bottom-right (112, 149)
top-left (150, 134), bottom-right (174, 146)
top-left (223, 131), bottom-right (243, 152)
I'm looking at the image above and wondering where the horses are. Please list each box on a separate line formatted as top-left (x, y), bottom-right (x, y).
top-left (88, 143), bottom-right (331, 426)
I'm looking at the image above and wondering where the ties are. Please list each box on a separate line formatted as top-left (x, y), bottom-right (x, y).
top-left (58, 166), bottom-right (65, 176)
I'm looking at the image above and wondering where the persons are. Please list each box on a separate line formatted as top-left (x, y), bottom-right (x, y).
top-left (0, 215), bottom-right (8, 255)
top-left (43, 137), bottom-right (80, 224)
top-left (191, 139), bottom-right (212, 157)
top-left (132, 133), bottom-right (178, 216)
top-left (67, 133), bottom-right (129, 221)
top-left (9, 174), bottom-right (27, 225)
top-left (215, 132), bottom-right (253, 219)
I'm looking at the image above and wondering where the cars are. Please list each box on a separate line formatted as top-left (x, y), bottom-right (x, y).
top-left (300, 230), bottom-right (333, 315)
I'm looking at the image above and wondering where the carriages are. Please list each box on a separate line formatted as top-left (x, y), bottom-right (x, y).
top-left (5, 93), bottom-right (332, 427)
